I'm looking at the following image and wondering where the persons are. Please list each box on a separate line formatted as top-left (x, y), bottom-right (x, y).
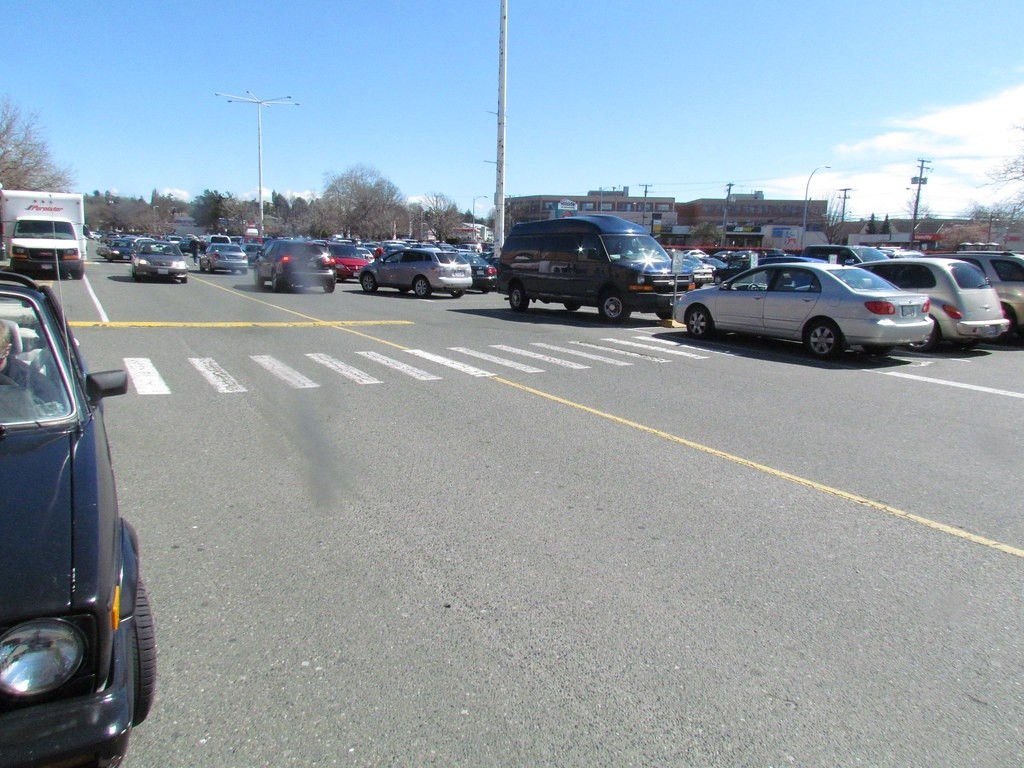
top-left (0, 320), bottom-right (61, 403)
top-left (199, 239), bottom-right (209, 254)
top-left (374, 245), bottom-right (384, 260)
top-left (189, 236), bottom-right (200, 266)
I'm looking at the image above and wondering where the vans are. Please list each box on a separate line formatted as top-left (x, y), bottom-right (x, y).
top-left (494, 212), bottom-right (695, 326)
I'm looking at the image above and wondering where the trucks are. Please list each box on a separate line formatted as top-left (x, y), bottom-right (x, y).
top-left (0, 189), bottom-right (87, 280)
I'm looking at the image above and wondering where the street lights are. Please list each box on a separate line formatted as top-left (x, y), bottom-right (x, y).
top-left (800, 165), bottom-right (832, 252)
top-left (472, 195), bottom-right (489, 242)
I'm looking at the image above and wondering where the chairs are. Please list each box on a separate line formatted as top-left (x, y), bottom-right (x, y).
top-left (767, 275), bottom-right (785, 291)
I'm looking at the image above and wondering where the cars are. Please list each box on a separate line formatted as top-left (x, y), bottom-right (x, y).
top-left (834, 256), bottom-right (1011, 352)
top-left (87, 231), bottom-right (501, 292)
top-left (198, 242), bottom-right (249, 275)
top-left (130, 239), bottom-right (190, 284)
top-left (0, 270), bottom-right (159, 768)
top-left (253, 239), bottom-right (340, 293)
top-left (672, 260), bottom-right (936, 361)
top-left (665, 243), bottom-right (1024, 333)
top-left (358, 247), bottom-right (474, 298)
